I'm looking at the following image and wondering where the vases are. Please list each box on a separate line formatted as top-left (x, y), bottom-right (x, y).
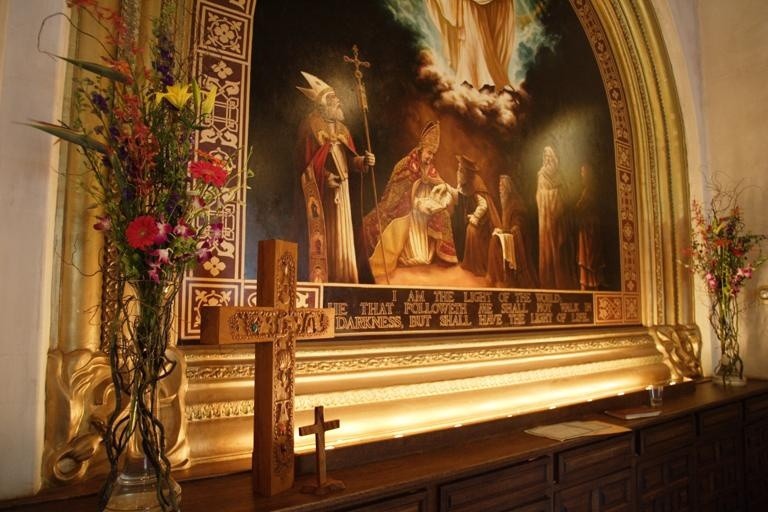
top-left (712, 316), bottom-right (750, 389)
top-left (103, 385), bottom-right (183, 503)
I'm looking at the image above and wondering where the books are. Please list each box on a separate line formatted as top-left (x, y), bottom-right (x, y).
top-left (604, 406), bottom-right (661, 421)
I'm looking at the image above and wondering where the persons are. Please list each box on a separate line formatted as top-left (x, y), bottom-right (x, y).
top-left (485, 174), bottom-right (539, 289)
top-left (363, 148), bottom-right (459, 278)
top-left (447, 155), bottom-right (502, 278)
top-left (300, 92), bottom-right (375, 285)
top-left (574, 159), bottom-right (607, 290)
top-left (535, 141), bottom-right (579, 291)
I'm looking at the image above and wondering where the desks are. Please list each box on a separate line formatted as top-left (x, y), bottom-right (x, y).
top-left (2, 373), bottom-right (766, 510)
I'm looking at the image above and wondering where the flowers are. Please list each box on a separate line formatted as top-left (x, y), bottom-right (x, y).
top-left (675, 170), bottom-right (762, 325)
top-left (41, 0), bottom-right (257, 386)
